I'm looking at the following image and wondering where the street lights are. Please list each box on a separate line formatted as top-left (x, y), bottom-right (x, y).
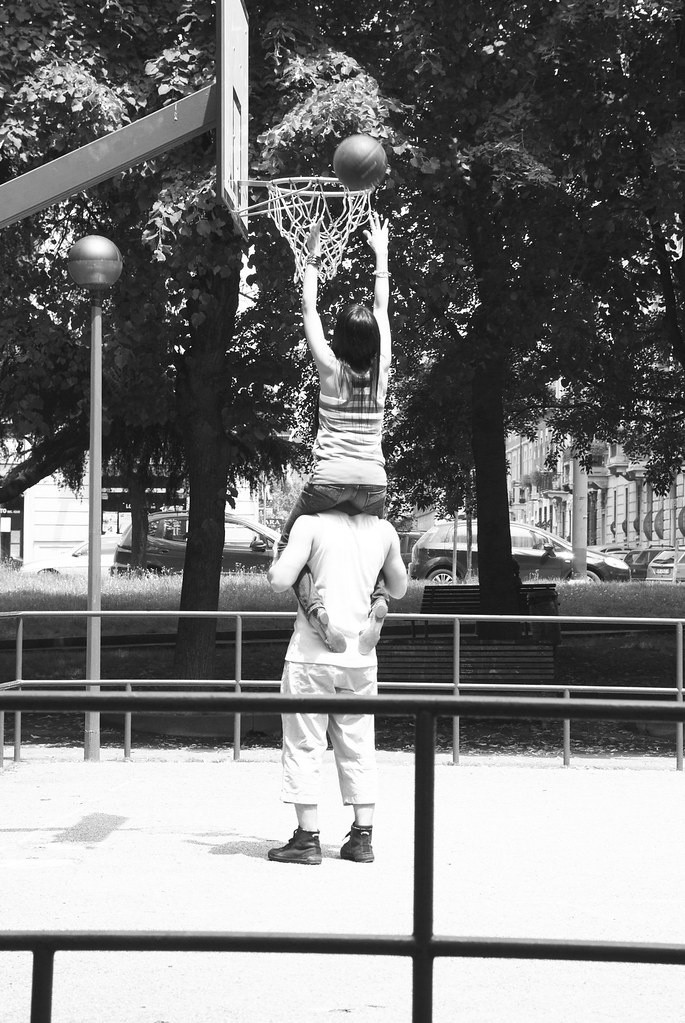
top-left (68, 235), bottom-right (123, 761)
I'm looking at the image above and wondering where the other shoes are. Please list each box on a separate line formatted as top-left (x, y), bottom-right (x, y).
top-left (312, 607), bottom-right (347, 652)
top-left (359, 597), bottom-right (388, 655)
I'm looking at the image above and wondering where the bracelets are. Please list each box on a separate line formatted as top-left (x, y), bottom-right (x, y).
top-left (306, 254), bottom-right (321, 266)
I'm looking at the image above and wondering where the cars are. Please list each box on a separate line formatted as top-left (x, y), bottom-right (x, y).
top-left (408, 521), bottom-right (630, 585)
top-left (22, 533), bottom-right (122, 583)
top-left (588, 543), bottom-right (685, 585)
top-left (109, 511), bottom-right (278, 579)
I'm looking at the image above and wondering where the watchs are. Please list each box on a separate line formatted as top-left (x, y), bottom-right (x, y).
top-left (372, 270), bottom-right (390, 278)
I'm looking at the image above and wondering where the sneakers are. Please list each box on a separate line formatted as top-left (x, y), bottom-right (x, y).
top-left (268, 826), bottom-right (322, 865)
top-left (340, 821), bottom-right (374, 863)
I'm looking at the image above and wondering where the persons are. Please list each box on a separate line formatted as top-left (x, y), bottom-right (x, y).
top-left (265, 500), bottom-right (408, 864)
top-left (276, 211), bottom-right (390, 655)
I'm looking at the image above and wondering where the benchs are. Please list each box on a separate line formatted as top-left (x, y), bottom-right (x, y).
top-left (375, 633), bottom-right (564, 748)
top-left (403, 579), bottom-right (560, 638)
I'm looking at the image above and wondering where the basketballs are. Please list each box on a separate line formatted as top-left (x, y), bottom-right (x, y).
top-left (332, 133), bottom-right (388, 189)
